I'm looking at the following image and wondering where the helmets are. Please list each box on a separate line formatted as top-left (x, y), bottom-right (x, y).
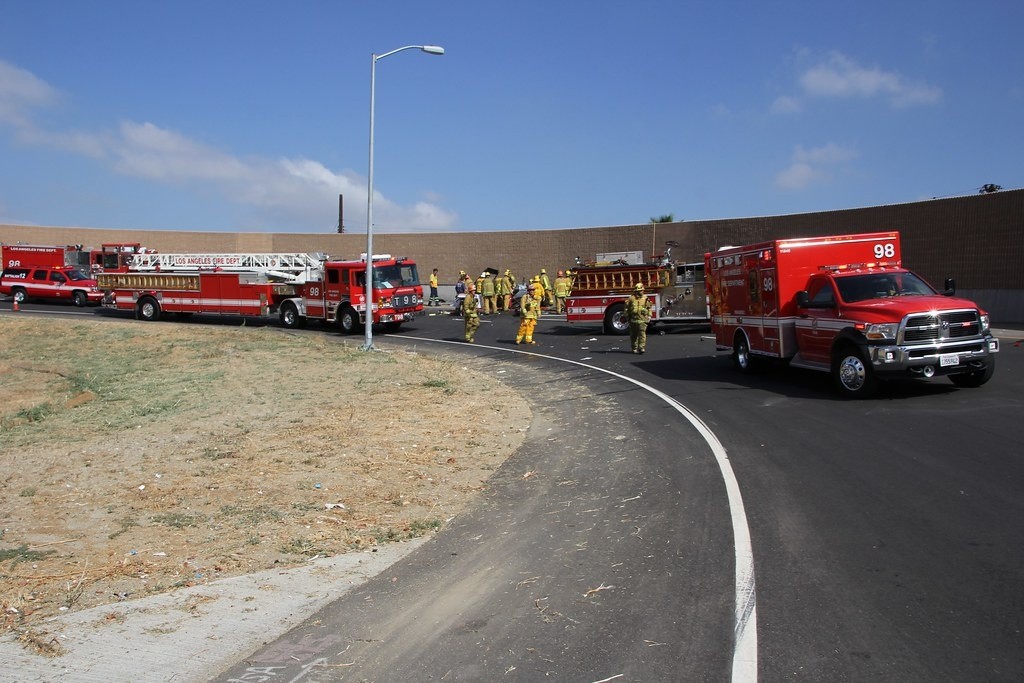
top-left (527, 284), bottom-right (535, 291)
top-left (634, 283), bottom-right (645, 291)
top-left (565, 270), bottom-right (571, 276)
top-left (503, 272), bottom-right (508, 276)
top-left (465, 274), bottom-right (469, 278)
top-left (533, 275), bottom-right (540, 281)
top-left (529, 278), bottom-right (533, 281)
top-left (486, 272), bottom-right (490, 275)
top-left (540, 269), bottom-right (546, 273)
top-left (506, 269), bottom-right (511, 273)
top-left (459, 270), bottom-right (465, 274)
top-left (480, 272), bottom-right (485, 277)
top-left (468, 284), bottom-right (476, 291)
top-left (557, 270), bottom-right (563, 277)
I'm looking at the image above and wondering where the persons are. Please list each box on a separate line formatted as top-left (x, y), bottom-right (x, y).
top-left (475, 272), bottom-right (485, 294)
top-left (515, 285), bottom-right (540, 344)
top-left (428, 268), bottom-right (441, 306)
top-left (481, 272), bottom-right (499, 314)
top-left (458, 271), bottom-right (474, 287)
top-left (539, 268), bottom-right (554, 306)
top-left (532, 275), bottom-right (545, 318)
top-left (455, 278), bottom-right (467, 316)
top-left (551, 270), bottom-right (567, 314)
top-left (565, 270), bottom-right (571, 278)
top-left (463, 285), bottom-right (480, 343)
top-left (501, 272), bottom-right (513, 312)
top-left (624, 283), bottom-right (652, 353)
top-left (527, 279), bottom-right (533, 295)
top-left (507, 269), bottom-right (516, 287)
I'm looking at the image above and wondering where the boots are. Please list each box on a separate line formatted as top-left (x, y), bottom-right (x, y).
top-left (428, 301), bottom-right (432, 306)
top-left (436, 302), bottom-right (441, 306)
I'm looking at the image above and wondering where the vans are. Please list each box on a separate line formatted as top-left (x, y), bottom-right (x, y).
top-left (0, 264), bottom-right (104, 307)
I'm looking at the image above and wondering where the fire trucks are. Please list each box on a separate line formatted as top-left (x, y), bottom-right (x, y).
top-left (704, 230), bottom-right (1000, 399)
top-left (0, 243), bottom-right (159, 307)
top-left (92, 253), bottom-right (423, 335)
top-left (566, 248), bottom-right (711, 335)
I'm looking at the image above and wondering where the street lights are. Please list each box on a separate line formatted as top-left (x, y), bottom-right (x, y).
top-left (364, 44), bottom-right (445, 345)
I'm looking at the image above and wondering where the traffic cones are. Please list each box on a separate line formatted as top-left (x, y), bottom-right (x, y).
top-left (12, 295), bottom-right (20, 312)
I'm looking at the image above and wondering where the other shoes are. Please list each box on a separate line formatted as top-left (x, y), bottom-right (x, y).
top-left (466, 338), bottom-right (474, 343)
top-left (516, 341), bottom-right (519, 345)
top-left (633, 349), bottom-right (645, 353)
top-left (526, 341), bottom-right (536, 345)
